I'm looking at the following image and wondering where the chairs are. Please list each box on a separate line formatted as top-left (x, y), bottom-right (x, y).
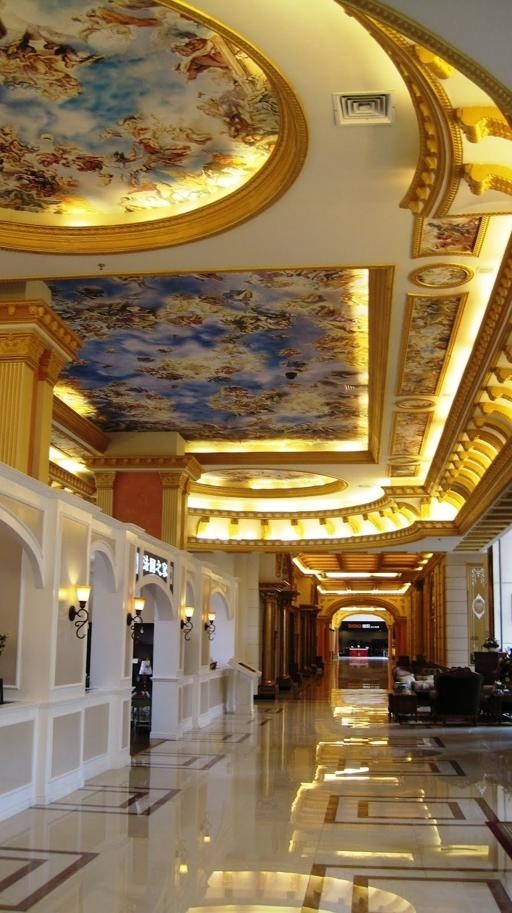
top-left (387, 661), bottom-right (512, 725)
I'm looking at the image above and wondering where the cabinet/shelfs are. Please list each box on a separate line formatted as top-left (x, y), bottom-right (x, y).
top-left (474, 652), bottom-right (508, 684)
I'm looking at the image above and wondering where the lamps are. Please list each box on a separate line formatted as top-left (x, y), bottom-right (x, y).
top-left (68, 586), bottom-right (91, 639)
top-left (181, 606), bottom-right (194, 641)
top-left (127, 598), bottom-right (145, 641)
top-left (138, 660), bottom-right (153, 697)
top-left (205, 611), bottom-right (216, 641)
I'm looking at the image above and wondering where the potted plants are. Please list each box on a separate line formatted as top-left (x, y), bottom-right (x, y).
top-left (483, 638), bottom-right (499, 652)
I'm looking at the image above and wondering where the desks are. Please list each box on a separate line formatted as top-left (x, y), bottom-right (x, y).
top-left (131, 696), bottom-right (152, 744)
